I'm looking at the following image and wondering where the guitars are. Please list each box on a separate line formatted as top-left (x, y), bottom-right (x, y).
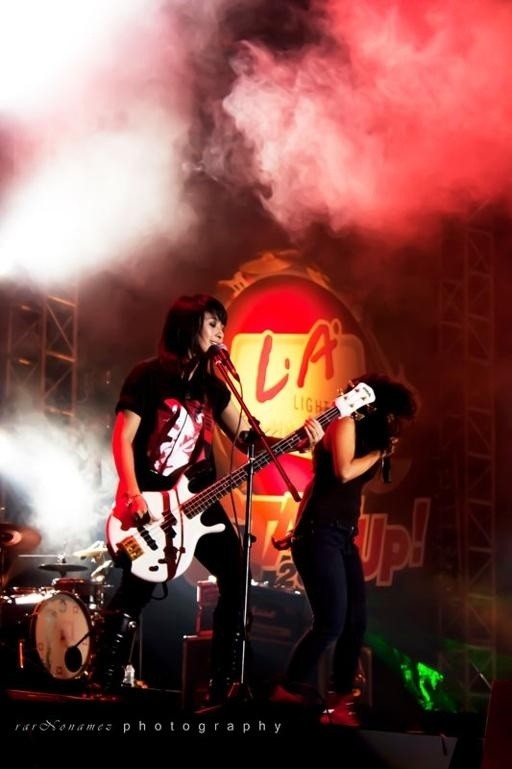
top-left (105, 375), bottom-right (377, 584)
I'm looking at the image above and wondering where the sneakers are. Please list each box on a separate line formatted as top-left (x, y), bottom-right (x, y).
top-left (270, 684), bottom-right (304, 704)
top-left (318, 693), bottom-right (360, 727)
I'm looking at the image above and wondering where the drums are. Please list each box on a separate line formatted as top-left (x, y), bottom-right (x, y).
top-left (51, 577), bottom-right (106, 612)
top-left (0, 587), bottom-right (94, 685)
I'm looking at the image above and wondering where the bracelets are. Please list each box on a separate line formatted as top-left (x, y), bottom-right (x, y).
top-left (126, 494), bottom-right (142, 509)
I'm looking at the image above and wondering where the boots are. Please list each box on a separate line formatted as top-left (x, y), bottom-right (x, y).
top-left (91, 609), bottom-right (140, 695)
top-left (209, 606), bottom-right (246, 705)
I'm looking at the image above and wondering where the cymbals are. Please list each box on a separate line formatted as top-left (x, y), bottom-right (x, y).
top-left (38, 563), bottom-right (88, 572)
top-left (18, 554), bottom-right (81, 559)
top-left (0, 523), bottom-right (42, 555)
top-left (77, 547), bottom-right (108, 557)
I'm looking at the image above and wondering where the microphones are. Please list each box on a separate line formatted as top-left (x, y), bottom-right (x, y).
top-left (214, 343), bottom-right (240, 381)
top-left (381, 432), bottom-right (392, 484)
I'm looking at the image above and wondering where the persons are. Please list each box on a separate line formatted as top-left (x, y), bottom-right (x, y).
top-left (78, 290), bottom-right (328, 702)
top-left (271, 373), bottom-right (421, 726)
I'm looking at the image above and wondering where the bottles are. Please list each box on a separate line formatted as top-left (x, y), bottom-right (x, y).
top-left (122, 662), bottom-right (135, 688)
